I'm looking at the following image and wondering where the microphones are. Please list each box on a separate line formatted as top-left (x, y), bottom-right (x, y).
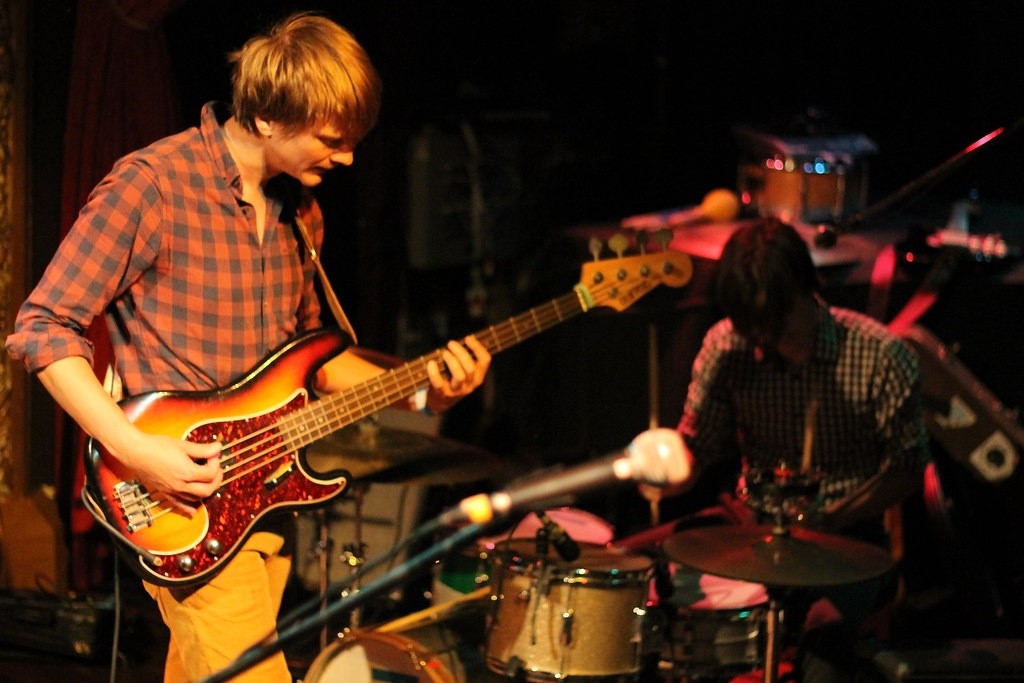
top-left (440, 427), bottom-right (692, 527)
top-left (536, 510), bottom-right (580, 563)
top-left (815, 227), bottom-right (837, 249)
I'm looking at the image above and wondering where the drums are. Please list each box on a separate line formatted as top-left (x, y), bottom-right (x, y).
top-left (656, 570), bottom-right (771, 666)
top-left (303, 617), bottom-right (476, 683)
top-left (473, 535), bottom-right (657, 683)
top-left (735, 133), bottom-right (878, 228)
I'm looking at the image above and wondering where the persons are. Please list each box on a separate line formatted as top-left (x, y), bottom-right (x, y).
top-left (5, 16), bottom-right (492, 683)
top-left (639, 219), bottom-right (930, 669)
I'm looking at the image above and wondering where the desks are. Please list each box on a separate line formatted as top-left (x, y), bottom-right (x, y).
top-left (574, 210), bottom-right (910, 427)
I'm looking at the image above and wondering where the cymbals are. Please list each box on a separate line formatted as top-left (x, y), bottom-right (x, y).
top-left (642, 219), bottom-right (878, 267)
top-left (661, 521), bottom-right (895, 589)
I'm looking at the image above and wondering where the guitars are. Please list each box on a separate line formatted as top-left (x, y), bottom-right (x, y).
top-left (81, 225), bottom-right (695, 589)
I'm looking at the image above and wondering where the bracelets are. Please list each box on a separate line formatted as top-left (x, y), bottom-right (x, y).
top-left (415, 387), bottom-right (435, 417)
